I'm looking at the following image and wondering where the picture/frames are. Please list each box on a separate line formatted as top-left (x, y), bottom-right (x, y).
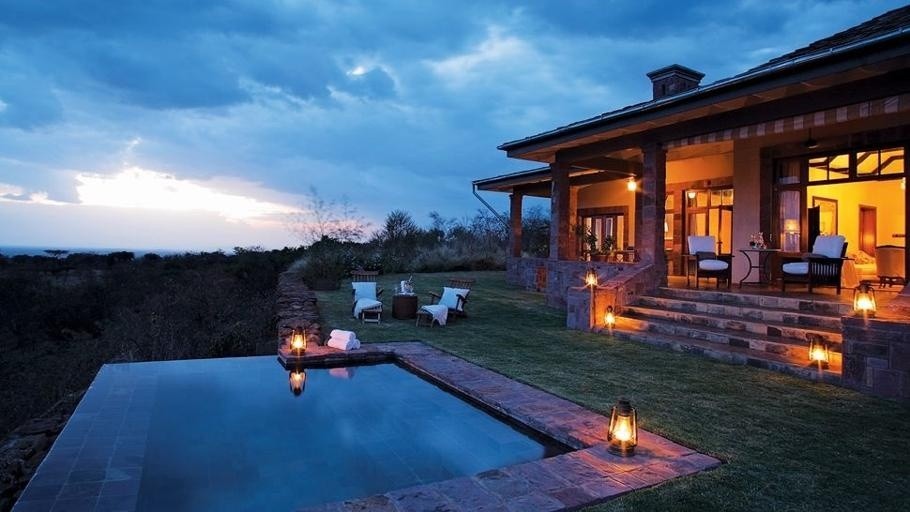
top-left (812, 196), bottom-right (838, 240)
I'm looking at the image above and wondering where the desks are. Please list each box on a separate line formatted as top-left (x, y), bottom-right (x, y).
top-left (391, 293), bottom-right (418, 321)
top-left (736, 248), bottom-right (786, 290)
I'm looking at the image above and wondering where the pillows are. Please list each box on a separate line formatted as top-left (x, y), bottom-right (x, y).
top-left (438, 286), bottom-right (470, 312)
top-left (800, 252), bottom-right (830, 264)
top-left (694, 251), bottom-right (716, 260)
top-left (351, 281), bottom-right (377, 301)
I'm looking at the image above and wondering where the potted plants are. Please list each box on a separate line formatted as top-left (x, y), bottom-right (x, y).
top-left (596, 235), bottom-right (615, 262)
top-left (583, 231), bottom-right (597, 261)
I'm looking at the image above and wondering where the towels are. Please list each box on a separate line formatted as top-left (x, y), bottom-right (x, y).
top-left (328, 329), bottom-right (361, 352)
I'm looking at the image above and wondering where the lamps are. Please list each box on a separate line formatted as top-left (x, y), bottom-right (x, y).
top-left (606, 396), bottom-right (638, 458)
top-left (289, 325), bottom-right (308, 356)
top-left (807, 333), bottom-right (832, 371)
top-left (604, 306), bottom-right (617, 329)
top-left (852, 281), bottom-right (877, 318)
top-left (288, 368), bottom-right (307, 395)
top-left (585, 267), bottom-right (598, 290)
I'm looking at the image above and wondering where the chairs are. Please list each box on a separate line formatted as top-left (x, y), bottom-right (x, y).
top-left (415, 278), bottom-right (478, 330)
top-left (781, 234), bottom-right (848, 296)
top-left (873, 244), bottom-right (905, 288)
top-left (682, 234), bottom-right (734, 291)
top-left (348, 269), bottom-right (385, 325)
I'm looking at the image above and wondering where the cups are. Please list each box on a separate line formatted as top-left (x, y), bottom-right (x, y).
top-left (749, 232), bottom-right (765, 249)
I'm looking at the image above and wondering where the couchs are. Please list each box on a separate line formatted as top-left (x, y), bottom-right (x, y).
top-left (849, 250), bottom-right (878, 275)
